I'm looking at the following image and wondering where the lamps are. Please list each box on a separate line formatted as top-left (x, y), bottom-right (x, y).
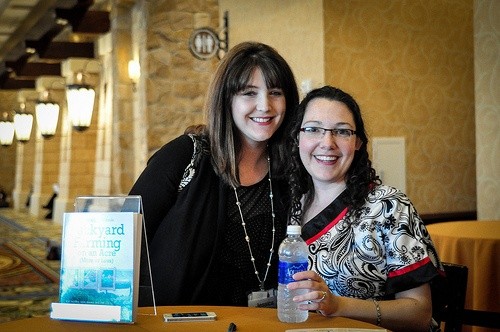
top-left (0, 57), bottom-right (107, 146)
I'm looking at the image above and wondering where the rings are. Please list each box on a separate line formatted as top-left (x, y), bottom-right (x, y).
top-left (319, 290), bottom-right (326, 300)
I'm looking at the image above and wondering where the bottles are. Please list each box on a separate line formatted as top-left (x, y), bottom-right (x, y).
top-left (276, 225), bottom-right (310, 323)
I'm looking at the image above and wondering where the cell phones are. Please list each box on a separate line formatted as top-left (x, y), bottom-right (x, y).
top-left (162, 311), bottom-right (217, 322)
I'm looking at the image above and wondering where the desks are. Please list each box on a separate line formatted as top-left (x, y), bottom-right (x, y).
top-left (426, 220), bottom-right (500, 312)
top-left (0, 306), bottom-right (390, 332)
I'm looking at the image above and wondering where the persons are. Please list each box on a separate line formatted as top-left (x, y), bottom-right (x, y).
top-left (284, 84), bottom-right (445, 332)
top-left (119, 41), bottom-right (300, 306)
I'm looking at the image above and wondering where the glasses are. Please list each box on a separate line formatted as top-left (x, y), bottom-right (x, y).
top-left (300, 125), bottom-right (358, 143)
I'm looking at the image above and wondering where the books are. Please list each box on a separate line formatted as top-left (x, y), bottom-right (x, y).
top-left (60, 211), bottom-right (142, 321)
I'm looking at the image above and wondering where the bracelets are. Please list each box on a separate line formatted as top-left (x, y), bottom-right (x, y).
top-left (374, 299), bottom-right (381, 326)
